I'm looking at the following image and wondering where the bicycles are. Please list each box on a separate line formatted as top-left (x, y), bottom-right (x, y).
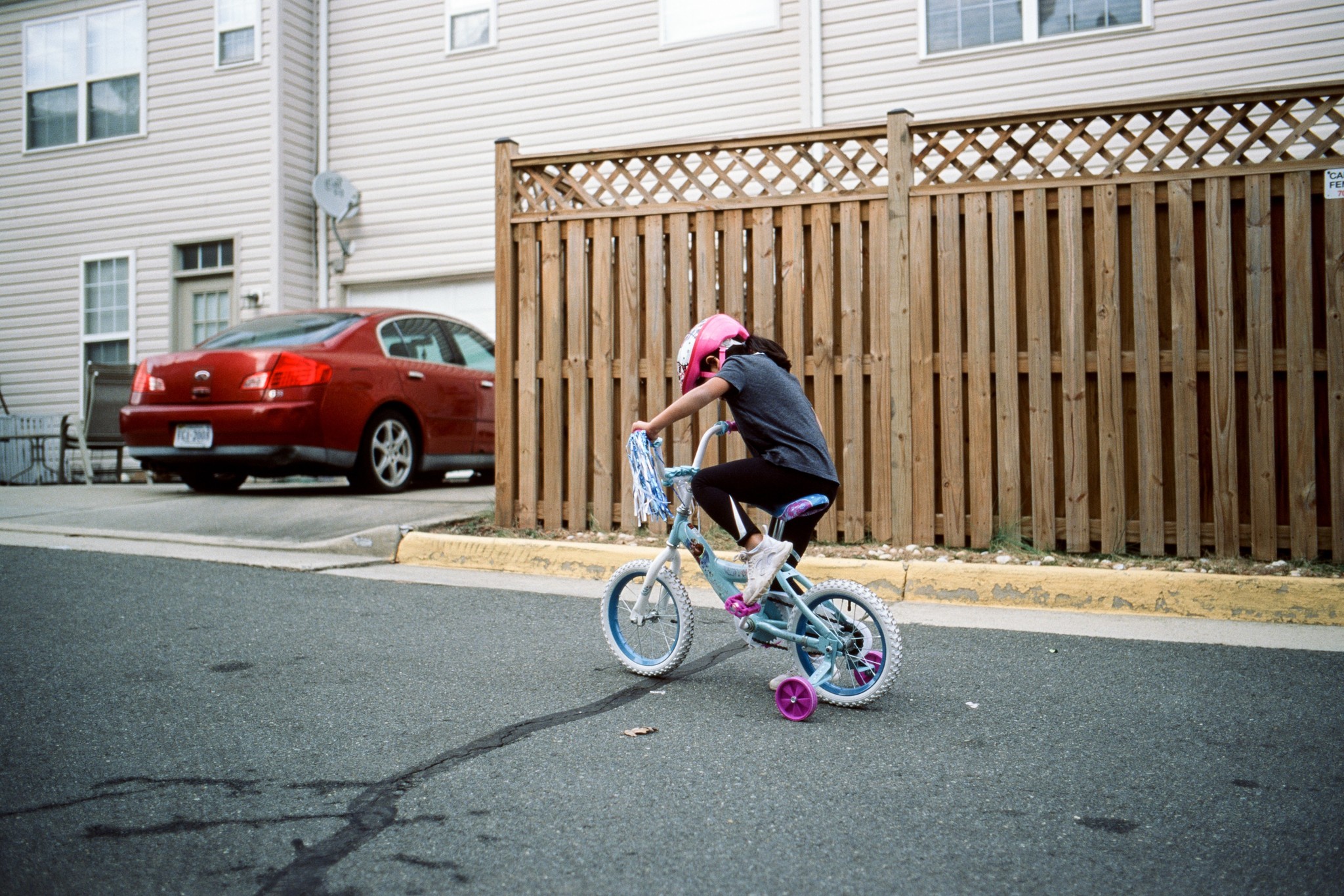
top-left (600, 422), bottom-right (902, 721)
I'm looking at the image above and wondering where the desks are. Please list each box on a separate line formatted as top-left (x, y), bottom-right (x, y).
top-left (0, 434), bottom-right (61, 487)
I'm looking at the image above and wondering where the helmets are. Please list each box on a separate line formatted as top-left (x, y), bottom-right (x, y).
top-left (676, 315), bottom-right (748, 398)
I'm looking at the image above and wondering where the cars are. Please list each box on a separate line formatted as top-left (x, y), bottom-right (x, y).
top-left (121, 308), bottom-right (497, 496)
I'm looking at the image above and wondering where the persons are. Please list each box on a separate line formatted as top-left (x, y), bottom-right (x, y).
top-left (631, 309), bottom-right (842, 614)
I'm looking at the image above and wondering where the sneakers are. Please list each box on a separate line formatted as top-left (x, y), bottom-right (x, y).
top-left (769, 653), bottom-right (839, 692)
top-left (738, 536), bottom-right (796, 604)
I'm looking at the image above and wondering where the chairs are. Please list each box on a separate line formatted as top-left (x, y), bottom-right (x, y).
top-left (59, 362), bottom-right (139, 478)
top-left (58, 371), bottom-right (135, 486)
top-left (389, 341), bottom-right (419, 358)
